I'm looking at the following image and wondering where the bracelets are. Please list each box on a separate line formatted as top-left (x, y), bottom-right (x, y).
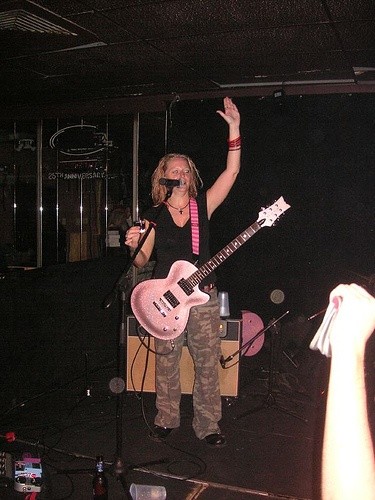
top-left (226, 136), bottom-right (242, 151)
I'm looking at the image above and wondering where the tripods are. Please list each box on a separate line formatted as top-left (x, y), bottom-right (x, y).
top-left (225, 310), bottom-right (308, 423)
top-left (50, 186), bottom-right (174, 500)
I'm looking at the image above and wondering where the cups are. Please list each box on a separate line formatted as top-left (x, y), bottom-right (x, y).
top-left (136, 221), bottom-right (146, 233)
top-left (129, 483), bottom-right (166, 500)
top-left (217, 291), bottom-right (230, 317)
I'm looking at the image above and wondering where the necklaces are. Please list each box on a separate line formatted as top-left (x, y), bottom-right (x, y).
top-left (166, 200), bottom-right (189, 215)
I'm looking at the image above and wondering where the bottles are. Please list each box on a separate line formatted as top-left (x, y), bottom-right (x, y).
top-left (92, 455), bottom-right (108, 500)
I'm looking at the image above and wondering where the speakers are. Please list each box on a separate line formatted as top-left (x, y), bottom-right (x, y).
top-left (126, 315), bottom-right (240, 397)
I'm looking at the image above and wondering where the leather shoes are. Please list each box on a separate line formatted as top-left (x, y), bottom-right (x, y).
top-left (204, 434), bottom-right (227, 447)
top-left (149, 426), bottom-right (175, 440)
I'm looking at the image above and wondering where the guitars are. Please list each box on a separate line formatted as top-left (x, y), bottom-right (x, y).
top-left (130, 195), bottom-right (291, 341)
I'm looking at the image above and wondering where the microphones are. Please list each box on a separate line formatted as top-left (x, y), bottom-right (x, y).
top-left (159, 178), bottom-right (184, 188)
top-left (220, 355), bottom-right (225, 367)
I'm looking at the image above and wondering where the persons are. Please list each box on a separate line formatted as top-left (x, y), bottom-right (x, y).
top-left (126, 95), bottom-right (243, 451)
top-left (318, 283), bottom-right (375, 500)
